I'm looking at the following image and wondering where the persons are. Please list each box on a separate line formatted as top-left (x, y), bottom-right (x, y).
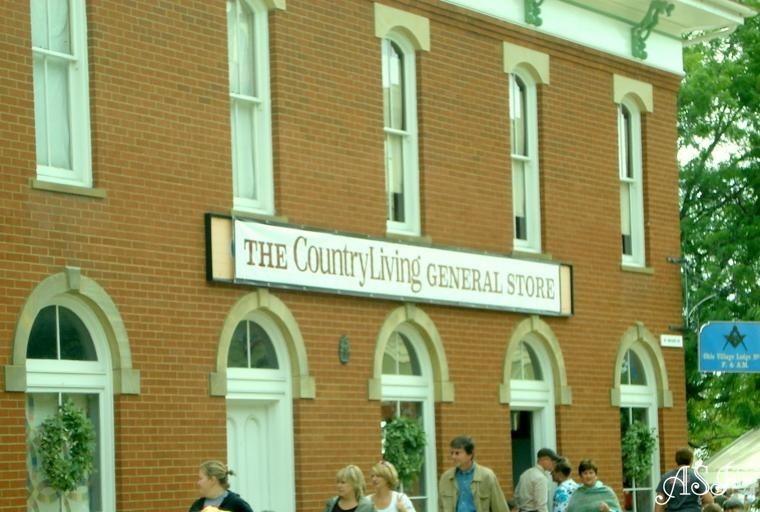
top-left (186, 459), bottom-right (252, 511)
top-left (512, 448), bottom-right (564, 511)
top-left (652, 448), bottom-right (715, 511)
top-left (323, 463), bottom-right (378, 511)
top-left (564, 459), bottom-right (625, 511)
top-left (361, 459), bottom-right (414, 511)
top-left (437, 435), bottom-right (510, 511)
top-left (550, 453), bottom-right (577, 511)
